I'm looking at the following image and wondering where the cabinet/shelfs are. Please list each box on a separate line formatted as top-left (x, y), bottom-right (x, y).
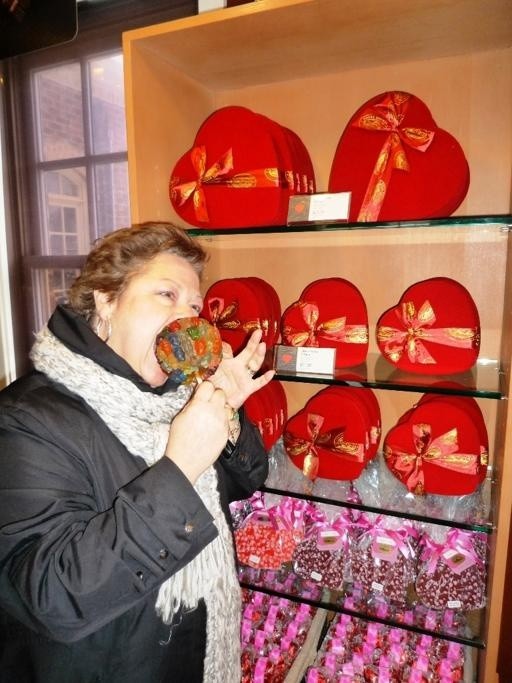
top-left (124, 0), bottom-right (512, 678)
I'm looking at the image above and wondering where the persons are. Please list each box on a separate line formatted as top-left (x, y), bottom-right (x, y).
top-left (0, 220), bottom-right (276, 683)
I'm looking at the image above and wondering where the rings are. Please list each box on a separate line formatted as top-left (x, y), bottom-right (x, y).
top-left (247, 366), bottom-right (256, 377)
top-left (224, 404), bottom-right (239, 422)
